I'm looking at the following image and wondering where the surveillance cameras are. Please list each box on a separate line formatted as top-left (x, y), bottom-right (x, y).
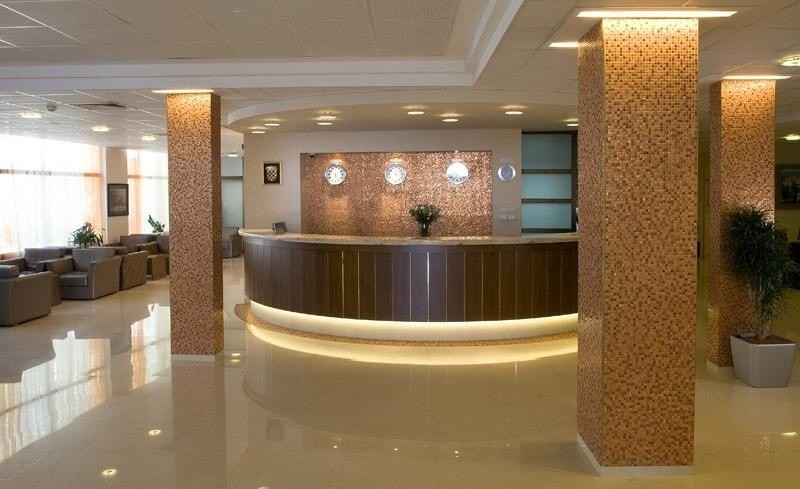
top-left (309, 153), bottom-right (314, 158)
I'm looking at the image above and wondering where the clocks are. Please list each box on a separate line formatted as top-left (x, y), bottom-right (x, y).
top-left (325, 163), bottom-right (347, 186)
top-left (448, 162), bottom-right (469, 185)
top-left (384, 163), bottom-right (407, 184)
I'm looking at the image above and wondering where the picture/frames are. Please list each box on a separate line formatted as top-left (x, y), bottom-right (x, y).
top-left (107, 184), bottom-right (129, 216)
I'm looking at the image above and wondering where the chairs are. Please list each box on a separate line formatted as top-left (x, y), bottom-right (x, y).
top-left (0, 233), bottom-right (170, 327)
top-left (220, 226), bottom-right (242, 259)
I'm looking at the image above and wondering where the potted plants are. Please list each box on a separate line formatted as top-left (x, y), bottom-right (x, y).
top-left (717, 205), bottom-right (800, 388)
top-left (409, 202), bottom-right (440, 237)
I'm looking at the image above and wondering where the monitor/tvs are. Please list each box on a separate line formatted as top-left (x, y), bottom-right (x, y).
top-left (272, 222), bottom-right (286, 232)
